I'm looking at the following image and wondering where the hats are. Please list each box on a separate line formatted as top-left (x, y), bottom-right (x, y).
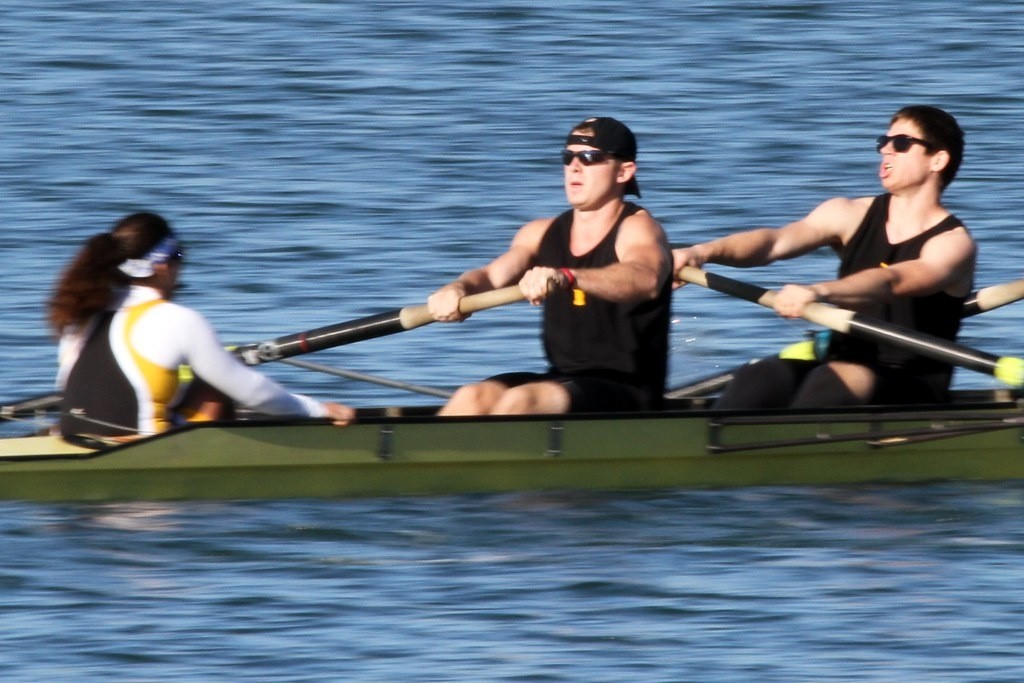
top-left (565, 117), bottom-right (641, 198)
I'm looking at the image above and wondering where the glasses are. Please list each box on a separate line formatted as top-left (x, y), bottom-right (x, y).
top-left (562, 150), bottom-right (623, 165)
top-left (876, 134), bottom-right (936, 153)
top-left (163, 251), bottom-right (183, 263)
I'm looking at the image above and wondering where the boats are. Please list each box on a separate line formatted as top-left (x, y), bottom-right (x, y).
top-left (0, 385), bottom-right (1024, 505)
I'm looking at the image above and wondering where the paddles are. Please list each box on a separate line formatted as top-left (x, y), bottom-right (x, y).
top-left (672, 264), bottom-right (1024, 389)
top-left (0, 275), bottom-right (558, 426)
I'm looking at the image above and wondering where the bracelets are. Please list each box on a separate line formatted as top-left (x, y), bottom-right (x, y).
top-left (560, 268), bottom-right (575, 290)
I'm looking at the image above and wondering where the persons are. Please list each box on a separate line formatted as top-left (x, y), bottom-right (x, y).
top-left (426, 116), bottom-right (675, 416)
top-left (670, 106), bottom-right (978, 409)
top-left (45, 212), bottom-right (355, 450)
